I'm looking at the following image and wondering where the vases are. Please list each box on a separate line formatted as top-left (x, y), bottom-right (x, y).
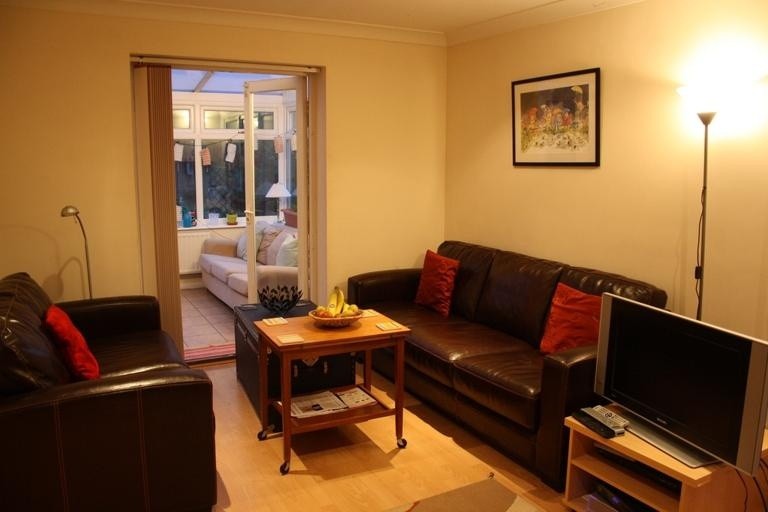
top-left (280, 208), bottom-right (298, 227)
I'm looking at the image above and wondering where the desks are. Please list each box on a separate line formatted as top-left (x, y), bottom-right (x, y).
top-left (253, 309), bottom-right (410, 475)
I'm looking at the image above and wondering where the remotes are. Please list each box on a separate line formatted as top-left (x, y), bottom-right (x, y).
top-left (580, 407), bottom-right (625, 435)
top-left (594, 405), bottom-right (629, 427)
top-left (571, 410), bottom-right (614, 439)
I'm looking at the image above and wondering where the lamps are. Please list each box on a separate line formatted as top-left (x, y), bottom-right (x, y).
top-left (265, 184), bottom-right (292, 223)
top-left (60, 206), bottom-right (95, 300)
top-left (690, 89), bottom-right (721, 321)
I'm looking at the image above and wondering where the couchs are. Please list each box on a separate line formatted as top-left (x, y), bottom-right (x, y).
top-left (200, 223), bottom-right (300, 310)
top-left (1, 272), bottom-right (215, 510)
top-left (346, 240), bottom-right (665, 493)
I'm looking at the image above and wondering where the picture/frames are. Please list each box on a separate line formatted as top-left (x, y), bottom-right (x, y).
top-left (511, 67), bottom-right (601, 168)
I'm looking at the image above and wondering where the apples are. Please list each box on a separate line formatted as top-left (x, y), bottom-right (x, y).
top-left (322, 312), bottom-right (332, 318)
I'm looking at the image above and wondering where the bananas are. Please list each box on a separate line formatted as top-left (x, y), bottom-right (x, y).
top-left (327, 287), bottom-right (344, 314)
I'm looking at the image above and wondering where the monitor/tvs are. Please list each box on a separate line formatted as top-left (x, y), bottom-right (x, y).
top-left (593, 292), bottom-right (768, 478)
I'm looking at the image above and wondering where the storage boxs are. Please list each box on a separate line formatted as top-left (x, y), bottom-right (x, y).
top-left (235, 299), bottom-right (356, 434)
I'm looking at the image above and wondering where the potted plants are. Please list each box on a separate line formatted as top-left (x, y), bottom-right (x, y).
top-left (226, 208), bottom-right (238, 225)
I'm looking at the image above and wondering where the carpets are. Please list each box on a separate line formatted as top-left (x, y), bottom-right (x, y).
top-left (378, 477), bottom-right (548, 512)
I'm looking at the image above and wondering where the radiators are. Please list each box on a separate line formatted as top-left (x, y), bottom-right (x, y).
top-left (177, 232), bottom-right (209, 275)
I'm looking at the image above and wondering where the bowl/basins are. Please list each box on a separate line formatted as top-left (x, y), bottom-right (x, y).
top-left (308, 309), bottom-right (365, 326)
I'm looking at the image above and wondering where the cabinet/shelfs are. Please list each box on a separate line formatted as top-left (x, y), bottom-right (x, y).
top-left (562, 403), bottom-right (767, 512)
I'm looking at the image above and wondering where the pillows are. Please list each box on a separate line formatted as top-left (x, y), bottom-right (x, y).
top-left (238, 227), bottom-right (266, 261)
top-left (414, 251), bottom-right (458, 319)
top-left (540, 282), bottom-right (602, 355)
top-left (42, 304), bottom-right (99, 380)
top-left (276, 235), bottom-right (299, 267)
top-left (257, 227), bottom-right (281, 265)
top-left (266, 230), bottom-right (289, 267)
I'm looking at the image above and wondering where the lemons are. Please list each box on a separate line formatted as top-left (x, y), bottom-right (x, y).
top-left (341, 311), bottom-right (356, 317)
top-left (317, 305), bottom-right (326, 310)
top-left (348, 304), bottom-right (359, 312)
top-left (344, 304), bottom-right (349, 311)
